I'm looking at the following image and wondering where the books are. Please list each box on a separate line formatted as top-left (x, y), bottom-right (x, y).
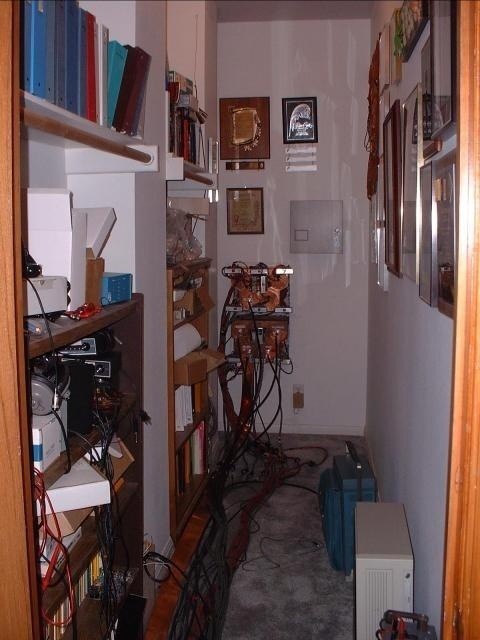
top-left (45, 552), bottom-right (107, 640)
top-left (170, 107), bottom-right (204, 168)
top-left (175, 421), bottom-right (204, 497)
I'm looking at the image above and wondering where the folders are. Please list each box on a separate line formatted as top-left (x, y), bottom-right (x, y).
top-left (21, 0), bottom-right (150, 136)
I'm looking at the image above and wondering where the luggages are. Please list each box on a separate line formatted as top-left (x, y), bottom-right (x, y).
top-left (320, 439), bottom-right (376, 570)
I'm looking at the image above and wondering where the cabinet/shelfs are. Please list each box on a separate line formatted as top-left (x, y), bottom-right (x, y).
top-left (20, 88), bottom-right (218, 638)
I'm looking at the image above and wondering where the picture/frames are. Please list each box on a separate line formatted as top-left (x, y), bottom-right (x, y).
top-left (381, 1), bottom-right (458, 320)
top-left (281, 96), bottom-right (319, 144)
top-left (226, 187), bottom-right (265, 234)
